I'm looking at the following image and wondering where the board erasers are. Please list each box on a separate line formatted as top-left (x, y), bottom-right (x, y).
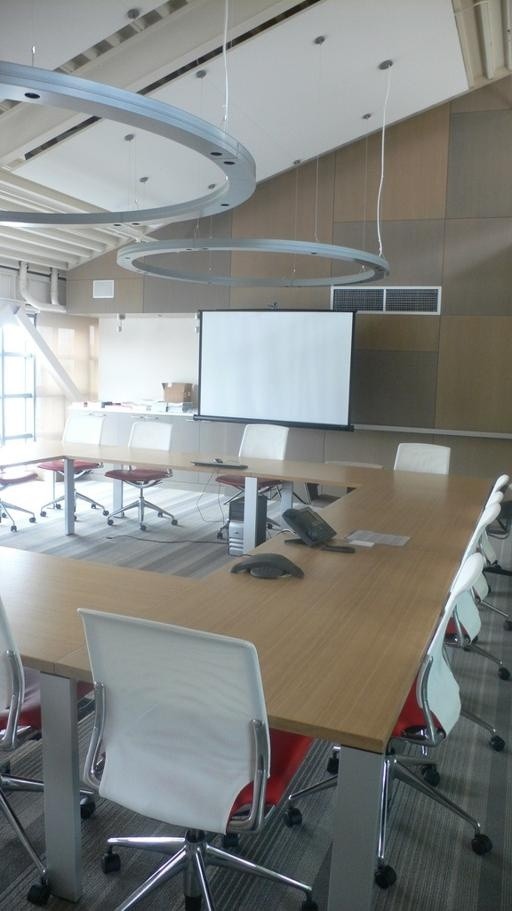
top-left (102, 402), bottom-right (112, 405)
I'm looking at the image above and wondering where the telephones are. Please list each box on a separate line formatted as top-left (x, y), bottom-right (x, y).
top-left (282, 507), bottom-right (337, 548)
top-left (229, 551), bottom-right (305, 581)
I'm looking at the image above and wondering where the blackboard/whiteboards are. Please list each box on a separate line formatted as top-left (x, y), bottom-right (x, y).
top-left (97, 317), bottom-right (201, 417)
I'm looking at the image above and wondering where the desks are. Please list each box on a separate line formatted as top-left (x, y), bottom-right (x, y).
top-left (2, 438), bottom-right (506, 755)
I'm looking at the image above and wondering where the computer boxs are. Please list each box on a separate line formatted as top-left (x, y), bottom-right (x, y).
top-left (228, 496), bottom-right (267, 556)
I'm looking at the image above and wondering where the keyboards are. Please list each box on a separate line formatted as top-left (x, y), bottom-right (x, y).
top-left (191, 460), bottom-right (248, 468)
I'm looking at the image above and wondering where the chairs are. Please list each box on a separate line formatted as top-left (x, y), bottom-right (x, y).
top-left (105, 420), bottom-right (179, 531)
top-left (1, 599), bottom-right (101, 910)
top-left (280, 549), bottom-right (493, 890)
top-left (216, 424), bottom-right (289, 539)
top-left (39, 414), bottom-right (110, 524)
top-left (1, 468), bottom-right (38, 533)
top-left (72, 604), bottom-right (319, 910)
top-left (394, 442), bottom-right (453, 474)
top-left (445, 471), bottom-right (512, 684)
